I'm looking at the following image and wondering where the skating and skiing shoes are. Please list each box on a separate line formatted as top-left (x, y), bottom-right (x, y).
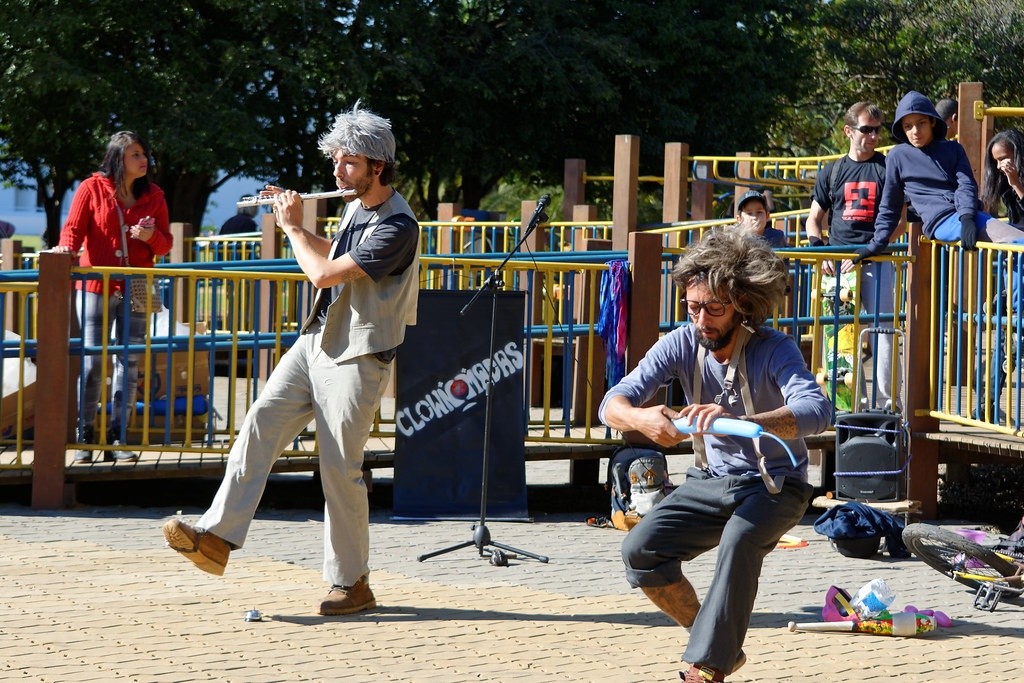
top-left (982, 291), bottom-right (1024, 374)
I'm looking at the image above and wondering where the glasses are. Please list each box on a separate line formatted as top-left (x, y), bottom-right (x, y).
top-left (680, 291), bottom-right (735, 317)
top-left (851, 126), bottom-right (882, 135)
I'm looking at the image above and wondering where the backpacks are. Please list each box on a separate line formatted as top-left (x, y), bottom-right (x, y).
top-left (609, 447), bottom-right (674, 531)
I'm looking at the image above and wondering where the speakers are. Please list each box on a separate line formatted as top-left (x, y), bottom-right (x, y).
top-left (834, 411), bottom-right (901, 504)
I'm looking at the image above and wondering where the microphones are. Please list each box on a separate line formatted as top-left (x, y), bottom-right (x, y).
top-left (525, 193), bottom-right (552, 234)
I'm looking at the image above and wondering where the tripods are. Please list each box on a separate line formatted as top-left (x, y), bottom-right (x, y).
top-left (418, 212), bottom-right (549, 563)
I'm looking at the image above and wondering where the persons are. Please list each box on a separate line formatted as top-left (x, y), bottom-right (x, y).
top-left (853, 89), bottom-right (1024, 350)
top-left (730, 191), bottom-right (789, 285)
top-left (935, 99), bottom-right (958, 141)
top-left (597, 222), bottom-right (836, 682)
top-left (215, 195), bottom-right (260, 248)
top-left (980, 129), bottom-right (1024, 229)
top-left (46, 131), bottom-right (173, 461)
top-left (164, 98), bottom-right (422, 615)
top-left (805, 100), bottom-right (910, 413)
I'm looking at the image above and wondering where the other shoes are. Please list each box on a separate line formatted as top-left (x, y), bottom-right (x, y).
top-left (74, 427), bottom-right (93, 463)
top-left (104, 435), bottom-right (138, 461)
top-left (962, 407), bottom-right (995, 426)
top-left (682, 649), bottom-right (748, 683)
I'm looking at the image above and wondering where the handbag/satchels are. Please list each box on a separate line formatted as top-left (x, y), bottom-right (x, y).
top-left (126, 277), bottom-right (163, 314)
top-left (813, 501), bottom-right (913, 559)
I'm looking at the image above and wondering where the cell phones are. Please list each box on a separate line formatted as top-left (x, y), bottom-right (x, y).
top-left (138, 218), bottom-right (156, 228)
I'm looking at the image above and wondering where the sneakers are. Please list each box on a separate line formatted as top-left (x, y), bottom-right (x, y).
top-left (163, 519), bottom-right (230, 576)
top-left (318, 584), bottom-right (376, 615)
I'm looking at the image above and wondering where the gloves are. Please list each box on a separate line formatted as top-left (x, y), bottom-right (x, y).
top-left (851, 248), bottom-right (878, 264)
top-left (960, 215), bottom-right (977, 250)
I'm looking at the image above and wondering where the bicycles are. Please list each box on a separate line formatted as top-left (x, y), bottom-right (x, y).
top-left (902, 522), bottom-right (1024, 615)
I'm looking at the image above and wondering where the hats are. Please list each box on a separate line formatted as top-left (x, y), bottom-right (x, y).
top-left (737, 190), bottom-right (766, 209)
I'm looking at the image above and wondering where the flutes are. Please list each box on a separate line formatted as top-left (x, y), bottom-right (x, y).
top-left (237, 189), bottom-right (358, 209)
